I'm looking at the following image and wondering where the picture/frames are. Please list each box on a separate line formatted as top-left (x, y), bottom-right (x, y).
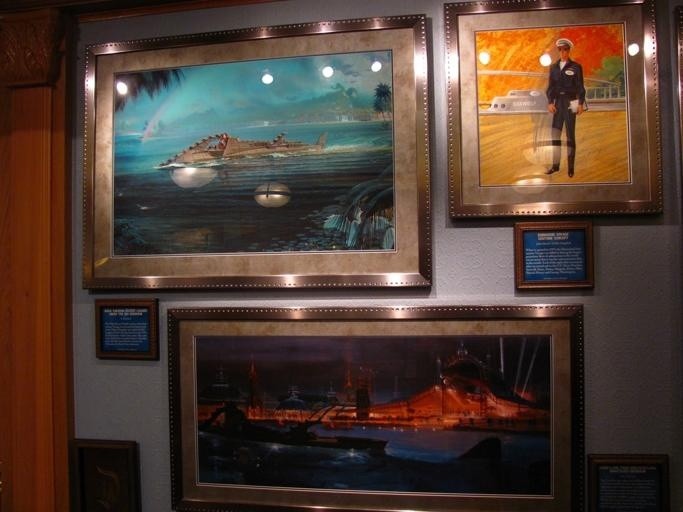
top-left (585, 455), bottom-right (673, 512)
top-left (169, 303), bottom-right (585, 511)
top-left (445, 3), bottom-right (667, 224)
top-left (512, 221), bottom-right (596, 293)
top-left (80, 11), bottom-right (434, 290)
top-left (93, 296), bottom-right (160, 363)
top-left (71, 438), bottom-right (143, 512)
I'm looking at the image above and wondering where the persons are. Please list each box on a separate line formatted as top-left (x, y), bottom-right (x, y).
top-left (545, 38), bottom-right (586, 179)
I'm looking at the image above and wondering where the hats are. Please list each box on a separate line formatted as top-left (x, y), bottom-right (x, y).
top-left (556, 38), bottom-right (573, 48)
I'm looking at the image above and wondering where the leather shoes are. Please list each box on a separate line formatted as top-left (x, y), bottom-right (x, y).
top-left (544, 167), bottom-right (559, 174)
top-left (568, 167), bottom-right (574, 177)
